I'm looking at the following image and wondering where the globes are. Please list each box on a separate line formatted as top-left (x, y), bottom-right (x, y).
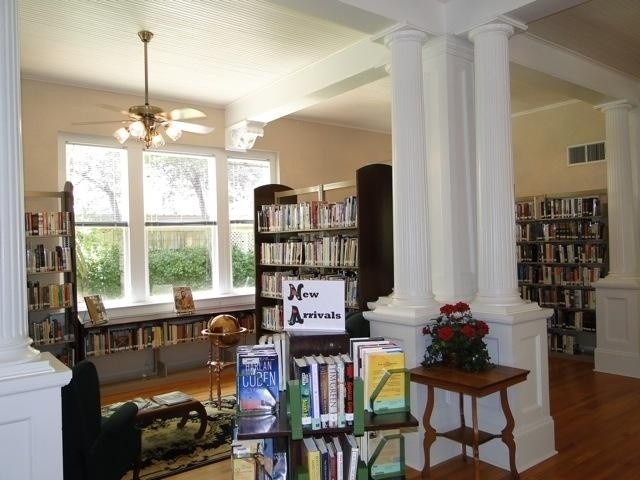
top-left (200, 314), bottom-right (249, 410)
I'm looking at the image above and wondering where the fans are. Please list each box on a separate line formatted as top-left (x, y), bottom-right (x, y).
top-left (70, 100), bottom-right (215, 136)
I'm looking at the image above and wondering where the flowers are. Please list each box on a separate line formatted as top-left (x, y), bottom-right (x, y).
top-left (418, 301), bottom-right (495, 373)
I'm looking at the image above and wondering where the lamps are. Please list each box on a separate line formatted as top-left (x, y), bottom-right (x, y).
top-left (113, 117), bottom-right (182, 149)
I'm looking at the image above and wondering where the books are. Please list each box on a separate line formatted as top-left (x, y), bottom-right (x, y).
top-left (84, 313), bottom-right (256, 352)
top-left (256, 204), bottom-right (358, 333)
top-left (108, 390), bottom-right (193, 410)
top-left (56, 348), bottom-right (74, 369)
top-left (173, 287), bottom-right (196, 314)
top-left (231, 333), bottom-right (290, 480)
top-left (26, 211), bottom-right (72, 236)
top-left (30, 314), bottom-right (64, 347)
top-left (288, 336), bottom-right (408, 480)
top-left (515, 197), bottom-right (601, 354)
top-left (27, 281), bottom-right (73, 310)
top-left (84, 295), bottom-right (109, 325)
top-left (27, 244), bottom-right (71, 273)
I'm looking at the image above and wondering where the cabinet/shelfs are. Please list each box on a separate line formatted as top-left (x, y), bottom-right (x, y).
top-left (237, 329), bottom-right (420, 480)
top-left (514, 188), bottom-right (610, 356)
top-left (25, 182), bottom-right (79, 368)
top-left (253, 163), bottom-right (392, 358)
top-left (78, 294), bottom-right (255, 385)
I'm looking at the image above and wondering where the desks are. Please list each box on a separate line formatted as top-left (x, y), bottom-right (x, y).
top-left (409, 361), bottom-right (530, 480)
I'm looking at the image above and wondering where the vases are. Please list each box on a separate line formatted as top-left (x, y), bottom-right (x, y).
top-left (446, 351), bottom-right (462, 368)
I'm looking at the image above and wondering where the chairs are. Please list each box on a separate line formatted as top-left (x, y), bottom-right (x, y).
top-left (61, 360), bottom-right (143, 480)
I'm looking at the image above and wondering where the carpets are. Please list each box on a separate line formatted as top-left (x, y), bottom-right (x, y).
top-left (102, 392), bottom-right (237, 480)
